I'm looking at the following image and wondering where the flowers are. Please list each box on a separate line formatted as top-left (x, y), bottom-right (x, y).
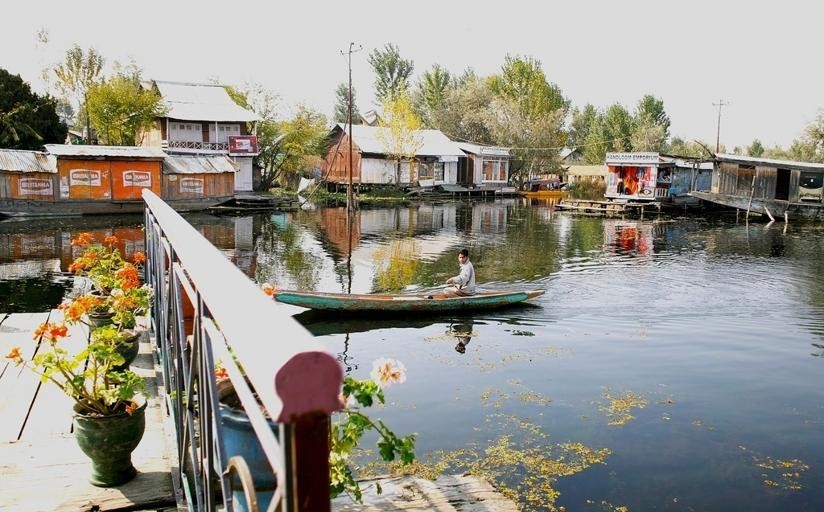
top-left (4, 287), bottom-right (154, 418)
top-left (239, 355), bottom-right (418, 504)
top-left (54, 232), bottom-right (151, 375)
top-left (211, 279), bottom-right (275, 386)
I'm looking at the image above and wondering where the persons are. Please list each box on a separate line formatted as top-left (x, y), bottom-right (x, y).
top-left (442, 249), bottom-right (475, 297)
top-left (446, 318), bottom-right (473, 354)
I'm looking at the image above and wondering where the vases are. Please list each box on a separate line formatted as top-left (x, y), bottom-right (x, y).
top-left (68, 399), bottom-right (147, 488)
top-left (208, 376), bottom-right (286, 512)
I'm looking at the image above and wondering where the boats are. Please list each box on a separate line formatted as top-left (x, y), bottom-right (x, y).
top-left (271, 289), bottom-right (546, 312)
top-left (689, 209), bottom-right (824, 265)
top-left (516, 178), bottom-right (572, 197)
top-left (685, 152), bottom-right (824, 221)
top-left (494, 186), bottom-right (516, 195)
top-left (525, 195), bottom-right (561, 204)
top-left (289, 301), bottom-right (543, 336)
